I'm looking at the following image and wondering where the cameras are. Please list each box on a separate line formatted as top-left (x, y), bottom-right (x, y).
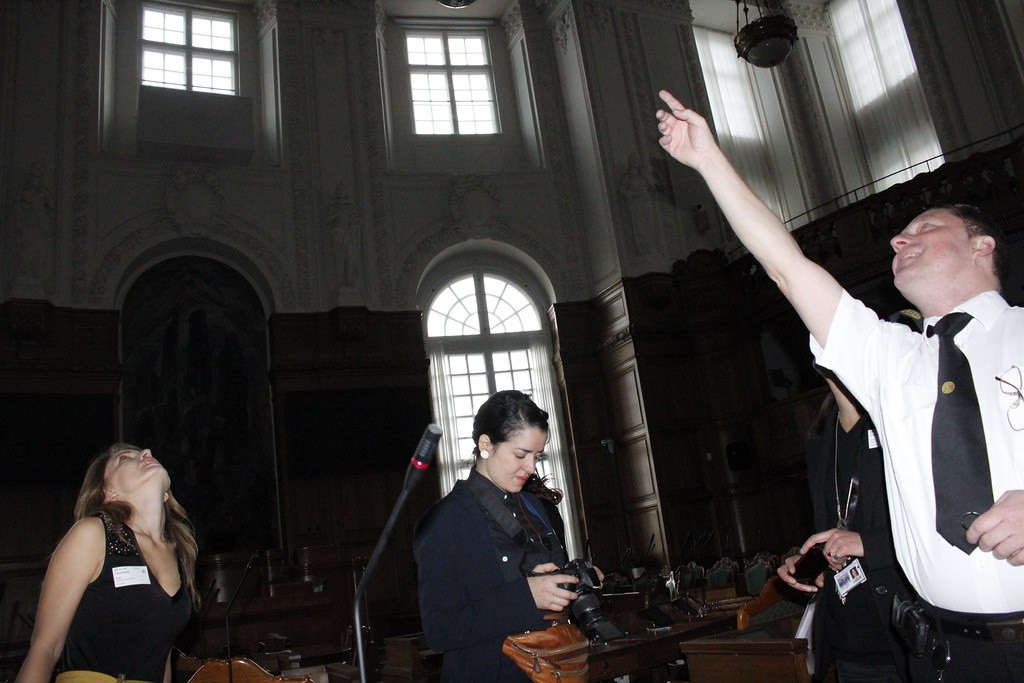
top-left (547, 559), bottom-right (606, 628)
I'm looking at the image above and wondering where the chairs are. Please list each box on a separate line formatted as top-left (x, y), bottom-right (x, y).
top-left (248, 631), bottom-right (292, 658)
top-left (743, 552), bottom-right (780, 596)
top-left (187, 656), bottom-right (315, 682)
top-left (737, 575), bottom-right (806, 630)
top-left (704, 556), bottom-right (741, 588)
top-left (685, 559), bottom-right (705, 588)
top-left (339, 624), bottom-right (371, 668)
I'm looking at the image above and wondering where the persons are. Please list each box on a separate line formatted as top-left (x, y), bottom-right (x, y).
top-left (656, 90), bottom-right (1024, 683)
top-left (851, 568), bottom-right (859, 579)
top-left (16, 443), bottom-right (203, 683)
top-left (774, 358), bottom-right (906, 680)
top-left (413, 390), bottom-right (604, 683)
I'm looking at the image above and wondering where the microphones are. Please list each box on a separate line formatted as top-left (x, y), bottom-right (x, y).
top-left (583, 528), bottom-right (763, 611)
top-left (6, 600), bottom-right (35, 656)
top-left (169, 424), bottom-right (444, 683)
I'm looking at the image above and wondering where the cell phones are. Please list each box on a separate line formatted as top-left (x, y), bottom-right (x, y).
top-left (788, 546), bottom-right (829, 584)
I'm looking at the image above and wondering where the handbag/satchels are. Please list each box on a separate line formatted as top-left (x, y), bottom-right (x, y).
top-left (503, 624), bottom-right (590, 683)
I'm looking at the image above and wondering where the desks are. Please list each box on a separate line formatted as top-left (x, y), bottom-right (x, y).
top-left (276, 640), bottom-right (352, 669)
top-left (587, 609), bottom-right (738, 683)
top-left (678, 612), bottom-right (811, 683)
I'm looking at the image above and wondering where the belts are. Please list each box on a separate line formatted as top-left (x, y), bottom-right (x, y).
top-left (915, 603), bottom-right (1024, 645)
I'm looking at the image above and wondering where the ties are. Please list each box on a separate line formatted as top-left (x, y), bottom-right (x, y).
top-left (925, 311), bottom-right (998, 554)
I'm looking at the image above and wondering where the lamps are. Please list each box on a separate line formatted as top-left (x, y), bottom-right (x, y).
top-left (734, 0), bottom-right (799, 69)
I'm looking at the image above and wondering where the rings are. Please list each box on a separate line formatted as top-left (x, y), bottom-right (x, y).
top-left (826, 551), bottom-right (834, 557)
top-left (834, 554), bottom-right (841, 561)
